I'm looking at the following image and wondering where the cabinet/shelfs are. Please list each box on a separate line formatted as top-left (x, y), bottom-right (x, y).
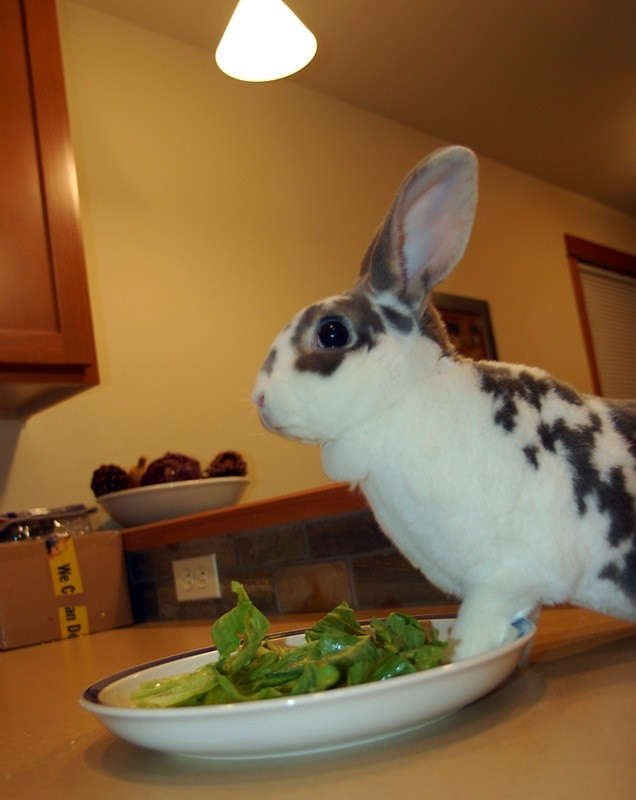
top-left (0, 0), bottom-right (101, 422)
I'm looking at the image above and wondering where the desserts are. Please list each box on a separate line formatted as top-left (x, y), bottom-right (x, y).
top-left (89, 450), bottom-right (248, 494)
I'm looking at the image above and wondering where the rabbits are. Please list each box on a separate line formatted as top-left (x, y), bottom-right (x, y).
top-left (252, 144), bottom-right (636, 664)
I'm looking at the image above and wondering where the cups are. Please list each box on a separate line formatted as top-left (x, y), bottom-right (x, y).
top-left (1, 514), bottom-right (97, 544)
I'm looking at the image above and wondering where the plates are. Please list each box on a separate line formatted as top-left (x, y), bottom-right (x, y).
top-left (98, 475), bottom-right (251, 527)
top-left (76, 613), bottom-right (538, 762)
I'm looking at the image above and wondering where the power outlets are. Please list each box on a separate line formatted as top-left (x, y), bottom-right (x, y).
top-left (173, 553), bottom-right (222, 600)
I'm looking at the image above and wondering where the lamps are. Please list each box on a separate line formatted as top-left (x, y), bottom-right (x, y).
top-left (215, 0), bottom-right (318, 84)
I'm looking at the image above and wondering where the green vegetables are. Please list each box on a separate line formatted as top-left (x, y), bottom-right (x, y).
top-left (127, 580), bottom-right (451, 710)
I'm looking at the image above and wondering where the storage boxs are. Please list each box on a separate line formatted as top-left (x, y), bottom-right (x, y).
top-left (0, 532), bottom-right (131, 652)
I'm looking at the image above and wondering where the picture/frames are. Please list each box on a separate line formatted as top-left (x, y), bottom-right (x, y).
top-left (431, 292), bottom-right (499, 362)
